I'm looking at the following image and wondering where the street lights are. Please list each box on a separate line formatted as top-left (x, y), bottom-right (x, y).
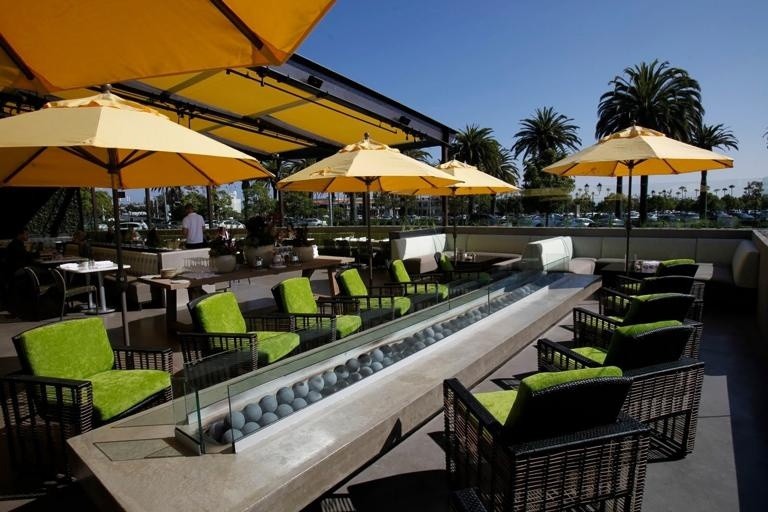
top-left (576, 181), bottom-right (736, 202)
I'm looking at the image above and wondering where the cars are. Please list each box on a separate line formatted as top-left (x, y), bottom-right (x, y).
top-left (91, 209), bottom-right (768, 233)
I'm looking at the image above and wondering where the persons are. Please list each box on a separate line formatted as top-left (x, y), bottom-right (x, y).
top-left (180, 201), bottom-right (207, 248)
top-left (4, 225), bottom-right (40, 264)
top-left (104, 227), bottom-right (115, 242)
top-left (123, 225), bottom-right (142, 241)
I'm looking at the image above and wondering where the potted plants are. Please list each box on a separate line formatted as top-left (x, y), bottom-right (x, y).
top-left (293, 224), bottom-right (317, 260)
top-left (240, 215), bottom-right (274, 267)
top-left (209, 226), bottom-right (235, 272)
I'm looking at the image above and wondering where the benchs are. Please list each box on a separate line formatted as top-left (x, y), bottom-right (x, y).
top-left (494, 235), bottom-right (562, 270)
top-left (705, 240), bottom-right (760, 320)
top-left (392, 234), bottom-right (454, 273)
top-left (534, 237), bottom-right (596, 275)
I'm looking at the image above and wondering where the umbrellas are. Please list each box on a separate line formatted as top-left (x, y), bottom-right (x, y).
top-left (0, 0), bottom-right (336, 99)
top-left (273, 132), bottom-right (466, 295)
top-left (541, 122), bottom-right (734, 269)
top-left (388, 158), bottom-right (522, 266)
top-left (0, 80), bottom-right (276, 368)
